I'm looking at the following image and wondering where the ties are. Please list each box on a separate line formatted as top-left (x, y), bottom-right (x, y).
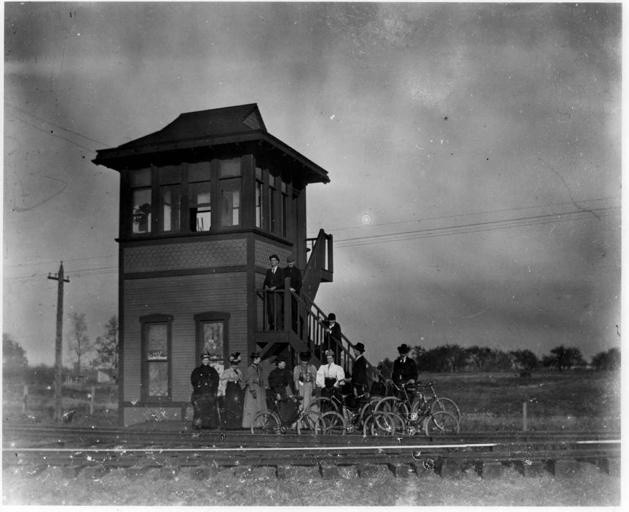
top-left (272, 269), bottom-right (275, 273)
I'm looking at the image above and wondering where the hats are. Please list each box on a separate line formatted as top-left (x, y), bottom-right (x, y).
top-left (397, 344), bottom-right (409, 351)
top-left (269, 255), bottom-right (279, 261)
top-left (352, 343), bottom-right (365, 351)
top-left (199, 353), bottom-right (210, 358)
top-left (275, 354), bottom-right (288, 362)
top-left (250, 351), bottom-right (263, 358)
top-left (287, 256), bottom-right (295, 263)
top-left (328, 313), bottom-right (335, 321)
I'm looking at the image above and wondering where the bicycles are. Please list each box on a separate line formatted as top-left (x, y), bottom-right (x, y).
top-left (250, 379), bottom-right (463, 438)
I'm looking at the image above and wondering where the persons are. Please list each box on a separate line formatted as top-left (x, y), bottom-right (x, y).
top-left (321, 311), bottom-right (344, 366)
top-left (191, 346), bottom-right (346, 431)
top-left (390, 342), bottom-right (419, 412)
top-left (261, 253), bottom-right (284, 332)
top-left (282, 255), bottom-right (302, 334)
top-left (348, 340), bottom-right (370, 406)
